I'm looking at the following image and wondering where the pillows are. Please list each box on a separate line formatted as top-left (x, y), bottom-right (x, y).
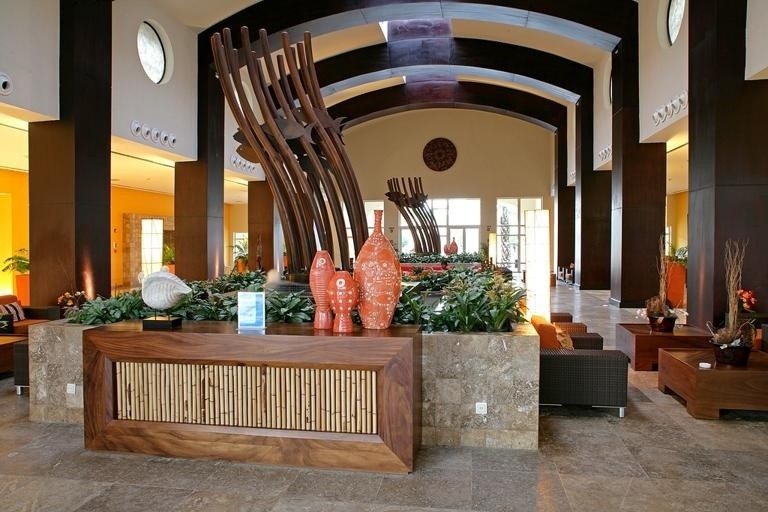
top-left (554, 322), bottom-right (575, 349)
top-left (0, 300), bottom-right (26, 321)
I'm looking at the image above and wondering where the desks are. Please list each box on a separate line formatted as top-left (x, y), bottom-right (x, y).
top-left (83, 321), bottom-right (421, 474)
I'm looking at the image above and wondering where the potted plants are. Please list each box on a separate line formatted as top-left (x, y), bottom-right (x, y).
top-left (645, 296), bottom-right (677, 333)
top-left (706, 321), bottom-right (753, 366)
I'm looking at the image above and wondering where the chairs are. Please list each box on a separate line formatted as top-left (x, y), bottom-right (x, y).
top-left (551, 313), bottom-right (587, 333)
top-left (0, 295), bottom-right (51, 334)
top-left (540, 333), bottom-right (631, 417)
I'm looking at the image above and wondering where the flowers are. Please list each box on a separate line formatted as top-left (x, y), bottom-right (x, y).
top-left (57, 291), bottom-right (85, 305)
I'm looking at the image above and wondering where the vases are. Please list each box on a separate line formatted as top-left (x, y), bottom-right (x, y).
top-left (327, 271), bottom-right (359, 333)
top-left (354, 210), bottom-right (402, 329)
top-left (311, 251), bottom-right (335, 330)
